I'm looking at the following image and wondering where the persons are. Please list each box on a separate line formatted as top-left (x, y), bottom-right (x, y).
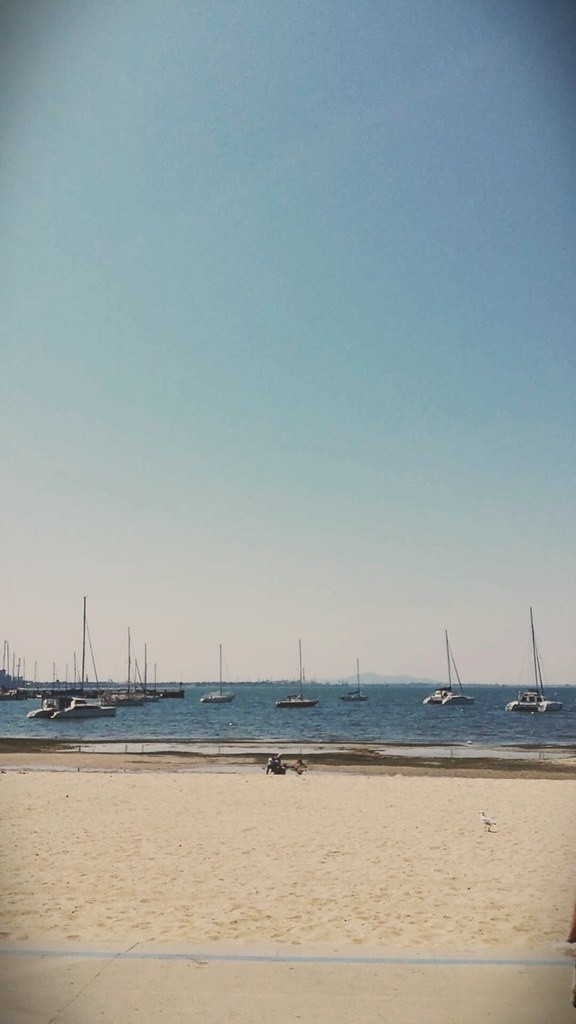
top-left (267, 754), bottom-right (286, 775)
top-left (566, 901), bottom-right (576, 1009)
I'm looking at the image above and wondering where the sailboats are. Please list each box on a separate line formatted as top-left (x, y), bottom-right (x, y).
top-left (504, 605), bottom-right (564, 714)
top-left (275, 638), bottom-right (320, 708)
top-left (339, 657), bottom-right (369, 702)
top-left (0, 594), bottom-right (165, 719)
top-left (421, 629), bottom-right (475, 707)
top-left (199, 644), bottom-right (236, 703)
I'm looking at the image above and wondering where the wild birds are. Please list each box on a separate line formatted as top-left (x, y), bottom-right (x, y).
top-left (477, 810), bottom-right (497, 832)
